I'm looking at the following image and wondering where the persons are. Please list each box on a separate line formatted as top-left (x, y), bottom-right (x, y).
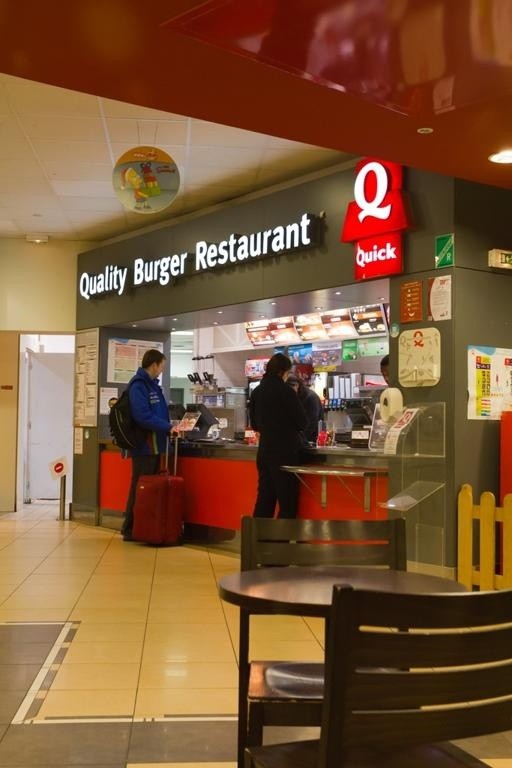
top-left (380, 355), bottom-right (389, 383)
top-left (121, 348), bottom-right (182, 540)
top-left (248, 352), bottom-right (311, 569)
top-left (284, 373), bottom-right (327, 447)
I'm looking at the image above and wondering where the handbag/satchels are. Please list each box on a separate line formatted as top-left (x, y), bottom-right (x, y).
top-left (108, 390), bottom-right (137, 450)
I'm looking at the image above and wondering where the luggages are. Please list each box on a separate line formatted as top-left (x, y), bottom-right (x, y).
top-left (131, 432), bottom-right (185, 545)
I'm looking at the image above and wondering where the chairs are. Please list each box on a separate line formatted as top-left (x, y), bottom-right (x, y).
top-left (240, 514), bottom-right (410, 746)
top-left (244, 583), bottom-right (512, 768)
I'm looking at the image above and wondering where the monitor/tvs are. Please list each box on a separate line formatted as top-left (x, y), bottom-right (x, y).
top-left (344, 399), bottom-right (380, 425)
top-left (167, 403), bottom-right (187, 421)
top-left (186, 403), bottom-right (219, 426)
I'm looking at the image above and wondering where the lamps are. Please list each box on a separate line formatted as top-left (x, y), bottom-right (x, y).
top-left (26, 236), bottom-right (48, 242)
